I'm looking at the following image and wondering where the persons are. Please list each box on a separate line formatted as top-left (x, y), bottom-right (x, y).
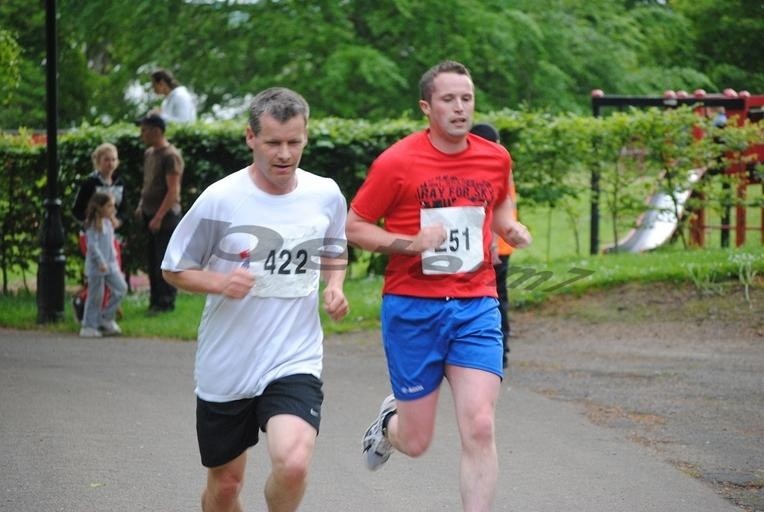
top-left (132, 113), bottom-right (186, 318)
top-left (468, 121), bottom-right (519, 370)
top-left (141, 69), bottom-right (196, 131)
top-left (158, 84), bottom-right (350, 512)
top-left (77, 189), bottom-right (128, 339)
top-left (344, 54), bottom-right (535, 512)
top-left (69, 143), bottom-right (135, 327)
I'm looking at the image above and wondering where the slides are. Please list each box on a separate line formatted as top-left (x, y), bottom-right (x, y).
top-left (601, 168), bottom-right (707, 252)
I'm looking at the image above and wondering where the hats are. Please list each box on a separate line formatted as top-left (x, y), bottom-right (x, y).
top-left (133, 115), bottom-right (166, 133)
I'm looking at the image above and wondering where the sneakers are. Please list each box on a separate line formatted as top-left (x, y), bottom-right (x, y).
top-left (361, 391), bottom-right (405, 474)
top-left (78, 327), bottom-right (104, 338)
top-left (100, 318), bottom-right (122, 335)
